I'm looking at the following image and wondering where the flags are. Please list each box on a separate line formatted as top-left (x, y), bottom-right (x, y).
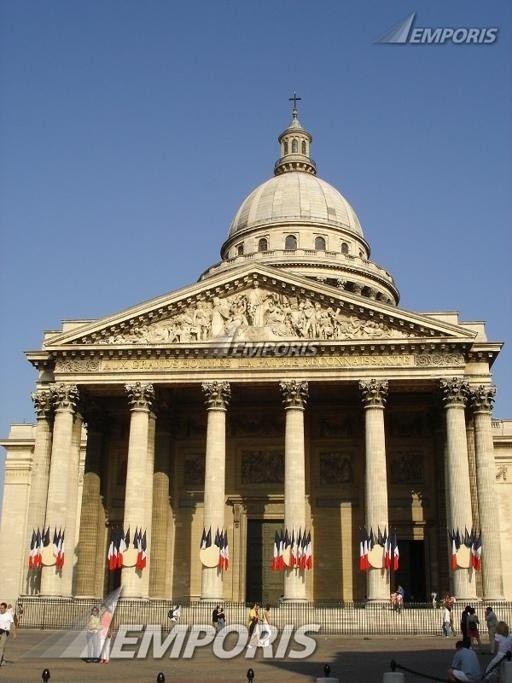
top-left (27, 524), bottom-right (66, 571)
top-left (271, 526), bottom-right (313, 574)
top-left (200, 526), bottom-right (229, 571)
top-left (359, 527), bottom-right (400, 571)
top-left (106, 524), bottom-right (148, 572)
top-left (448, 526), bottom-right (482, 573)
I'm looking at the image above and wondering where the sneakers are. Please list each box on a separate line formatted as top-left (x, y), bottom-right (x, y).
top-left (86, 657), bottom-right (109, 664)
top-left (443, 634), bottom-right (457, 639)
top-left (480, 650), bottom-right (495, 655)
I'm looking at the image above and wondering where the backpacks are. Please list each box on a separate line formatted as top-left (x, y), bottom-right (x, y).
top-left (167, 609), bottom-right (177, 618)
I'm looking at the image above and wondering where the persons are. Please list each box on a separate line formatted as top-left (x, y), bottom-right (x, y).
top-left (212, 604), bottom-right (220, 629)
top-left (84, 606), bottom-right (101, 663)
top-left (259, 602), bottom-right (276, 648)
top-left (390, 581), bottom-right (512, 683)
top-left (247, 602), bottom-right (262, 648)
top-left (217, 607), bottom-right (227, 631)
top-left (7, 603), bottom-right (14, 617)
top-left (170, 603), bottom-right (182, 632)
top-left (0, 603), bottom-right (17, 666)
top-left (96, 603), bottom-right (114, 664)
